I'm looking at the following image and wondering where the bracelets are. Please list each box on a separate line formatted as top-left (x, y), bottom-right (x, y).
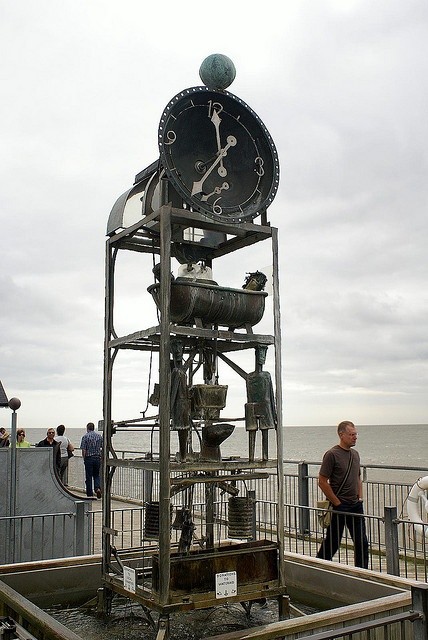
top-left (358, 496), bottom-right (362, 499)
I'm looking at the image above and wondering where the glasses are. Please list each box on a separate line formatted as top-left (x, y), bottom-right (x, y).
top-left (49, 432), bottom-right (55, 434)
top-left (18, 433), bottom-right (25, 436)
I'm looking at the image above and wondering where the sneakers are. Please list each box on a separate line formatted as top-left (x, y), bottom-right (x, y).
top-left (96, 489), bottom-right (101, 498)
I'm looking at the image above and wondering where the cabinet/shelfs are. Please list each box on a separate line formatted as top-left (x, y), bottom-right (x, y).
top-left (101, 205), bottom-right (290, 638)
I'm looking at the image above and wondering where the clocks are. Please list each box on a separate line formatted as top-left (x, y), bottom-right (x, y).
top-left (157, 87), bottom-right (280, 224)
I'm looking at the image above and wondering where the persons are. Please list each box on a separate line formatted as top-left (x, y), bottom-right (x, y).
top-left (315, 421), bottom-right (369, 570)
top-left (80, 422), bottom-right (102, 499)
top-left (8, 427), bottom-right (30, 449)
top-left (0, 427), bottom-right (10, 448)
top-left (37, 427), bottom-right (61, 477)
top-left (54, 424), bottom-right (75, 486)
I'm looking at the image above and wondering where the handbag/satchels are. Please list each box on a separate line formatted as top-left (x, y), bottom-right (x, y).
top-left (316, 501), bottom-right (332, 529)
top-left (67, 446), bottom-right (73, 459)
